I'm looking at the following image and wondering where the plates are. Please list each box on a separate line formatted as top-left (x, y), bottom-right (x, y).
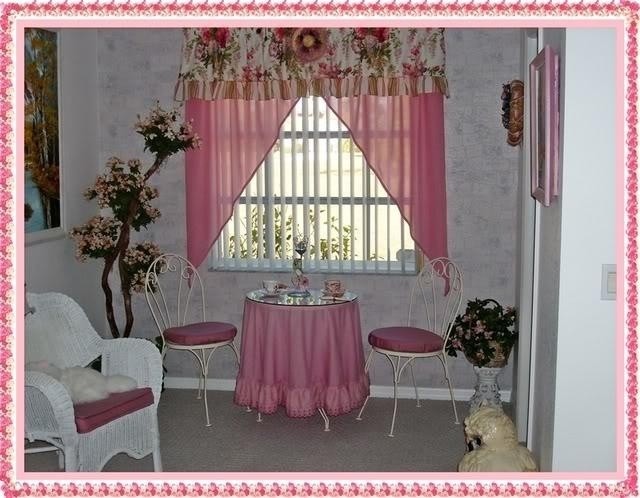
top-left (320, 288), bottom-right (345, 297)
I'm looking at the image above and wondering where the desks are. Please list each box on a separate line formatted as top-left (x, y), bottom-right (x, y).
top-left (240, 286), bottom-right (360, 430)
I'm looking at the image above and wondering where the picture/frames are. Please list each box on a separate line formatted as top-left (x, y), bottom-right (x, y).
top-left (24, 27), bottom-right (66, 245)
top-left (526, 44), bottom-right (561, 208)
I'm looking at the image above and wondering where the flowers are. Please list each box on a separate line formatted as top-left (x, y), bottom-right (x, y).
top-left (446, 296), bottom-right (518, 369)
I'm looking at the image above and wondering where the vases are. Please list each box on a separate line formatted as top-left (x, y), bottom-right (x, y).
top-left (468, 365), bottom-right (505, 412)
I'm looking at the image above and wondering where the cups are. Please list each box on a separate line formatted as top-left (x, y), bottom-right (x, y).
top-left (263, 280), bottom-right (278, 294)
top-left (324, 280), bottom-right (341, 292)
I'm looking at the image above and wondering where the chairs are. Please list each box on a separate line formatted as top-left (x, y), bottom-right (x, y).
top-left (356, 256), bottom-right (463, 438)
top-left (25, 291), bottom-right (167, 472)
top-left (145, 252), bottom-right (254, 428)
